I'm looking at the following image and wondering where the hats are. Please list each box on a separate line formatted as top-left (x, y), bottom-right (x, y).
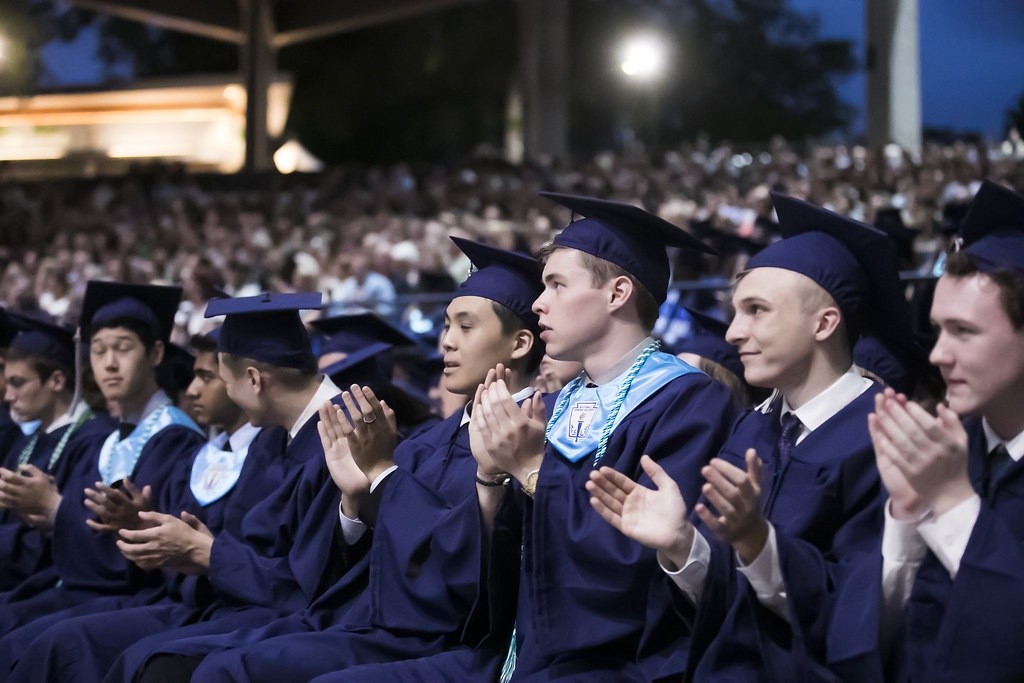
top-left (204, 290), bottom-right (324, 367)
top-left (69, 280), bottom-right (185, 415)
top-left (537, 190), bottom-right (720, 307)
top-left (744, 189), bottom-right (900, 344)
top-left (949, 178), bottom-right (1024, 278)
top-left (448, 230), bottom-right (548, 335)
top-left (0, 307), bottom-right (77, 363)
top-left (317, 342), bottom-right (394, 398)
top-left (307, 311), bottom-right (416, 374)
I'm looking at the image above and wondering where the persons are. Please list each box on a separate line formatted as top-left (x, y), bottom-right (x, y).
top-left (0, 292), bottom-right (361, 683)
top-left (0, 280), bottom-right (207, 630)
top-left (586, 192), bottom-right (906, 683)
top-left (308, 194), bottom-right (739, 682)
top-left (134, 236), bottom-right (548, 683)
top-left (823, 178), bottom-right (1024, 683)
top-left (0, 131), bottom-right (1024, 586)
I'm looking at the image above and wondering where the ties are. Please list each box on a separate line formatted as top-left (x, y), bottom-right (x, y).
top-left (777, 415), bottom-right (802, 472)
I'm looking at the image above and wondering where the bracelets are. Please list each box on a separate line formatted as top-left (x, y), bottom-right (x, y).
top-left (475, 475), bottom-right (512, 486)
top-left (476, 468), bottom-right (509, 477)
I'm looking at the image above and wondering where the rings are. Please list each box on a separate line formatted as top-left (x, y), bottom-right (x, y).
top-left (364, 415), bottom-right (377, 424)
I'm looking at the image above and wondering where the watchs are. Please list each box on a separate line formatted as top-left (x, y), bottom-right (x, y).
top-left (521, 470), bottom-right (539, 497)
